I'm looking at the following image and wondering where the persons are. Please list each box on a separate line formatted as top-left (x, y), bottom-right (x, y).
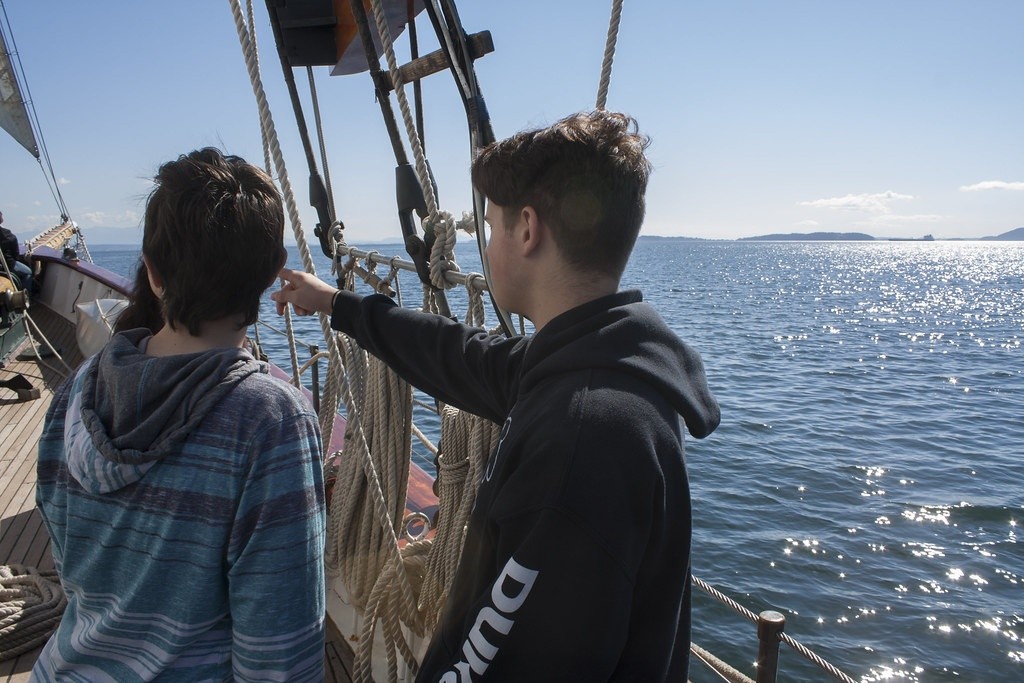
top-left (0, 211), bottom-right (19, 272)
top-left (271, 110), bottom-right (723, 683)
top-left (26, 146), bottom-right (330, 683)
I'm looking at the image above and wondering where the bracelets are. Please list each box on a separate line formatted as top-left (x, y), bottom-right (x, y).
top-left (331, 288), bottom-right (341, 306)
